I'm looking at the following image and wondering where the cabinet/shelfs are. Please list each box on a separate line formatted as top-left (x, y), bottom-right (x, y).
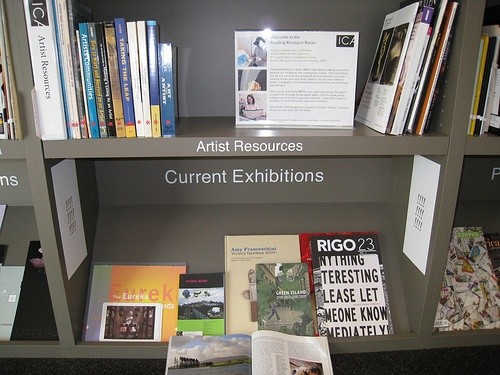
top-left (0, 0), bottom-right (500, 359)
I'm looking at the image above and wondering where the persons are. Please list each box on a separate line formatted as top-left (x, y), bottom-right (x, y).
top-left (248, 70), bottom-right (267, 91)
top-left (252, 37), bottom-right (267, 68)
top-left (244, 95), bottom-right (259, 121)
top-left (268, 301), bottom-right (281, 322)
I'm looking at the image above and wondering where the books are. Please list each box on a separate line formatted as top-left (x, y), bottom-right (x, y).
top-left (233, 0), bottom-right (500, 136)
top-left (0, 0), bottom-right (180, 140)
top-left (0, 203), bottom-right (500, 375)
